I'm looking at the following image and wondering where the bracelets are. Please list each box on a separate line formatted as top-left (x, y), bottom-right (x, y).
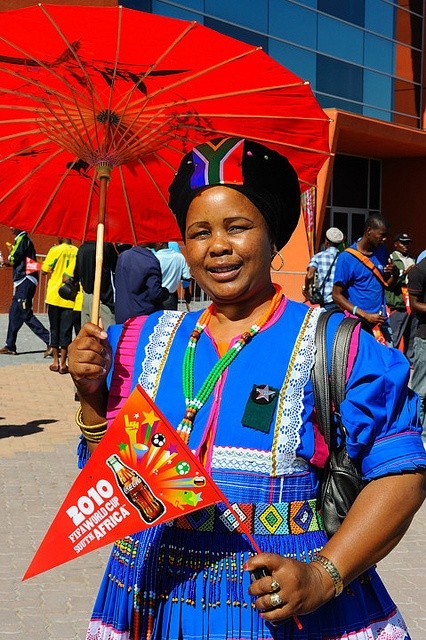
top-left (313, 554), bottom-right (348, 598)
top-left (351, 306), bottom-right (357, 315)
top-left (74, 407), bottom-right (107, 441)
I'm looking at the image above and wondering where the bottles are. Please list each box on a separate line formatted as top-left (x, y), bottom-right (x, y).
top-left (105, 454), bottom-right (165, 524)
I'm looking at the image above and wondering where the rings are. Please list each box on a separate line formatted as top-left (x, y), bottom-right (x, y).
top-left (270, 593), bottom-right (282, 607)
top-left (269, 577), bottom-right (282, 591)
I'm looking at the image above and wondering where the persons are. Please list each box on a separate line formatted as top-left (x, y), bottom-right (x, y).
top-left (415, 250), bottom-right (426, 263)
top-left (1, 227), bottom-right (52, 358)
top-left (332, 216), bottom-right (399, 348)
top-left (408, 258), bottom-right (426, 400)
top-left (385, 232), bottom-right (417, 369)
top-left (67, 138), bottom-right (426, 639)
top-left (302, 228), bottom-right (344, 310)
top-left (73, 241), bottom-right (118, 332)
top-left (41, 237), bottom-right (84, 376)
top-left (155, 243), bottom-right (193, 310)
top-left (114, 244), bottom-right (169, 325)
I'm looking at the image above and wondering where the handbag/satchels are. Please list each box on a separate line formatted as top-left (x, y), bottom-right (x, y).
top-left (315, 307), bottom-right (368, 539)
top-left (58, 277), bottom-right (78, 301)
top-left (311, 252), bottom-right (340, 304)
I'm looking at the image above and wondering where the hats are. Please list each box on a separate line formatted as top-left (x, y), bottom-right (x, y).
top-left (169, 136), bottom-right (300, 252)
top-left (326, 227), bottom-right (344, 244)
top-left (393, 231), bottom-right (413, 242)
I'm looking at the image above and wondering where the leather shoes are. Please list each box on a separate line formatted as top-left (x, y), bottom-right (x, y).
top-left (0, 347), bottom-right (16, 355)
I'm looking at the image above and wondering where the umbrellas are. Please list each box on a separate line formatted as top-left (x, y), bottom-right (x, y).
top-left (0, 2), bottom-right (333, 243)
